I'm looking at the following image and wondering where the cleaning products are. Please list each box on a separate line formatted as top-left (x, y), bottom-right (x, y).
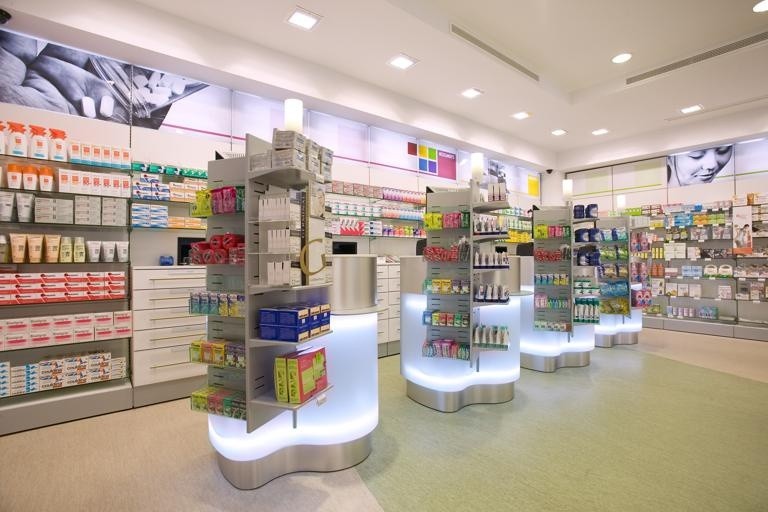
top-left (0, 118), bottom-right (68, 161)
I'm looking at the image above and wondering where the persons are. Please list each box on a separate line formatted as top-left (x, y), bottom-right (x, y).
top-left (666, 146), bottom-right (732, 187)
top-left (0, 30), bottom-right (151, 124)
top-left (734, 224), bottom-right (749, 248)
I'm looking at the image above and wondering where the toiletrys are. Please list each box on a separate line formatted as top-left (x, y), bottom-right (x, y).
top-left (0, 192), bottom-right (129, 263)
